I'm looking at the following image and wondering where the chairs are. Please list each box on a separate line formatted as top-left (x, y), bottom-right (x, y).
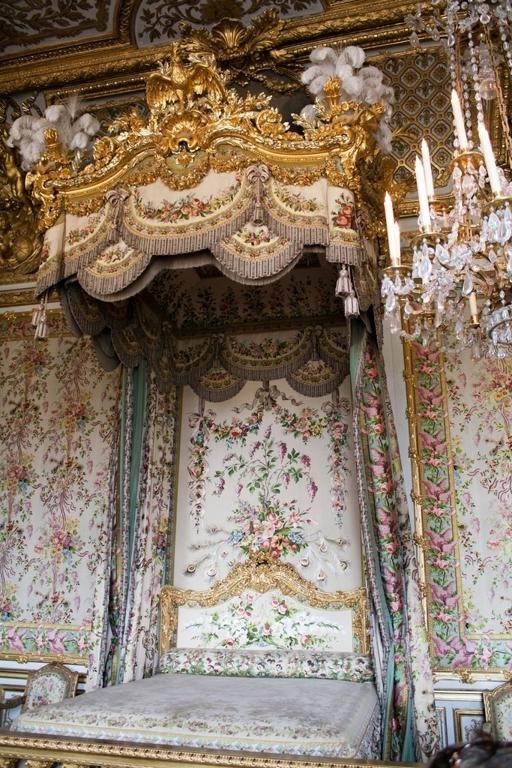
top-left (0, 661), bottom-right (79, 728)
top-left (482, 675), bottom-right (512, 744)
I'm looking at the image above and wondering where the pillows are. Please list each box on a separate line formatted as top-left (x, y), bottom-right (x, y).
top-left (157, 648), bottom-right (375, 683)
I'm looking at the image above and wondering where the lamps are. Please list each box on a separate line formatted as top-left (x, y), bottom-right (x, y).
top-left (377, 1), bottom-right (511, 359)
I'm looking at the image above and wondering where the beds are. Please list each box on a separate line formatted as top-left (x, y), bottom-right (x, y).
top-left (0, 31), bottom-right (445, 768)
